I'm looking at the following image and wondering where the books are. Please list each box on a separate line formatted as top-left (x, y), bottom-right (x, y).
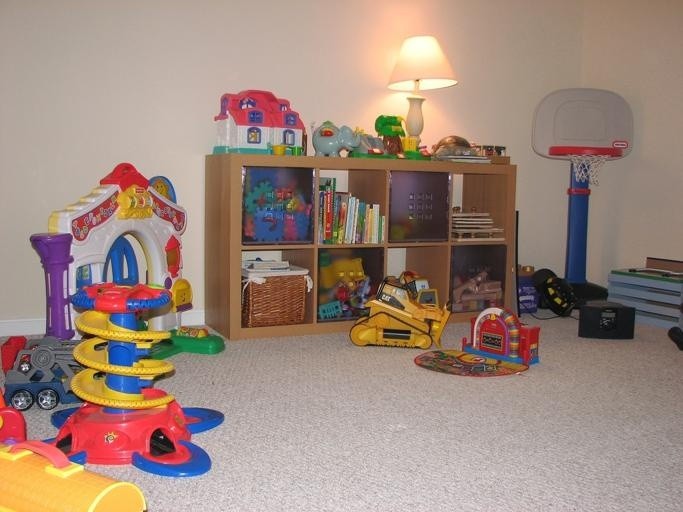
top-left (241, 258), bottom-right (309, 278)
top-left (319, 176), bottom-right (385, 244)
top-left (451, 212), bottom-right (506, 242)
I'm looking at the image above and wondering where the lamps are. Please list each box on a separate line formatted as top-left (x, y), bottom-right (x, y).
top-left (387, 35), bottom-right (458, 147)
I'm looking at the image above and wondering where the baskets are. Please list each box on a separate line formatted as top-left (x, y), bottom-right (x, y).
top-left (242, 266), bottom-right (309, 329)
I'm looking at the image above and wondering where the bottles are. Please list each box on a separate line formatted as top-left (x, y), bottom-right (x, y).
top-left (472, 144), bottom-right (506, 156)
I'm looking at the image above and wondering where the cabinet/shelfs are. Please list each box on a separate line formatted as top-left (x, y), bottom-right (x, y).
top-left (206, 155), bottom-right (516, 341)
